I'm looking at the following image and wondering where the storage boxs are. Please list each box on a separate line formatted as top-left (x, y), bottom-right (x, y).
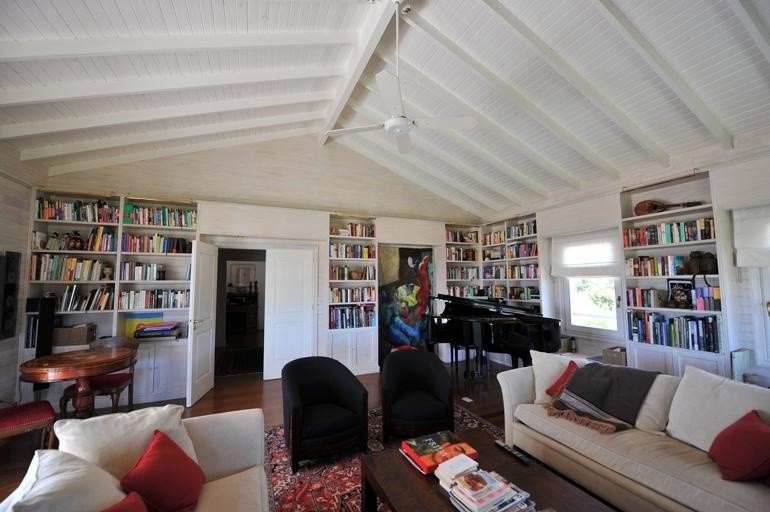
top-left (52, 323), bottom-right (96, 345)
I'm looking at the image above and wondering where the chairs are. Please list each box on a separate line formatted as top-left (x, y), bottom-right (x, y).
top-left (281, 356), bottom-right (368, 475)
top-left (60, 336), bottom-right (140, 419)
top-left (379, 348), bottom-right (454, 446)
top-left (0, 400), bottom-right (57, 449)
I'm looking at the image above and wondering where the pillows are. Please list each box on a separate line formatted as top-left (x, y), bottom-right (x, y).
top-left (0, 404), bottom-right (206, 512)
top-left (529, 350), bottom-right (770, 483)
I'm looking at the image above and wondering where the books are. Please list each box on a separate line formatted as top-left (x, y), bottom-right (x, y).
top-left (57, 285), bottom-right (114, 311)
top-left (35, 195), bottom-right (120, 225)
top-left (330, 221), bottom-right (376, 259)
top-left (446, 220), bottom-right (539, 301)
top-left (622, 219), bottom-right (723, 354)
top-left (32, 225), bottom-right (117, 253)
top-left (30, 255), bottom-right (105, 280)
top-left (119, 288), bottom-right (190, 310)
top-left (123, 203), bottom-right (196, 228)
top-left (330, 264), bottom-right (376, 303)
top-left (186, 264), bottom-right (191, 280)
top-left (401, 429), bottom-right (536, 511)
top-left (121, 232), bottom-right (191, 253)
top-left (329, 304), bottom-right (376, 328)
top-left (121, 259), bottom-right (165, 280)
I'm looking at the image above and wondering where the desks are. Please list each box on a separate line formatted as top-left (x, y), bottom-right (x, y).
top-left (20, 348), bottom-right (137, 418)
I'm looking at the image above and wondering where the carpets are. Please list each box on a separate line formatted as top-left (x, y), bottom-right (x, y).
top-left (264, 403), bottom-right (527, 511)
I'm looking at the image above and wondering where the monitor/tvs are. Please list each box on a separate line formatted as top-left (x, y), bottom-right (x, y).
top-left (0, 250), bottom-right (22, 341)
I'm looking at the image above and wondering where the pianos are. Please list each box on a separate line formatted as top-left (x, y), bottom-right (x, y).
top-left (422, 293), bottom-right (562, 379)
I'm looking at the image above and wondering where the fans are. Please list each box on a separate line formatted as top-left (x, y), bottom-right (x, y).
top-left (324, 0), bottom-right (478, 155)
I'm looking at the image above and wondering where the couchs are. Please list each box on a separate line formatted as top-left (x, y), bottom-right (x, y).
top-left (497, 360), bottom-right (770, 512)
top-left (182, 408), bottom-right (269, 512)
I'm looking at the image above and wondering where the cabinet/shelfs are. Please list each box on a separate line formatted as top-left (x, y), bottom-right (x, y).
top-left (618, 168), bottom-right (732, 376)
top-left (444, 211), bottom-right (542, 317)
top-left (329, 214), bottom-right (378, 375)
top-left (26, 187), bottom-right (196, 411)
